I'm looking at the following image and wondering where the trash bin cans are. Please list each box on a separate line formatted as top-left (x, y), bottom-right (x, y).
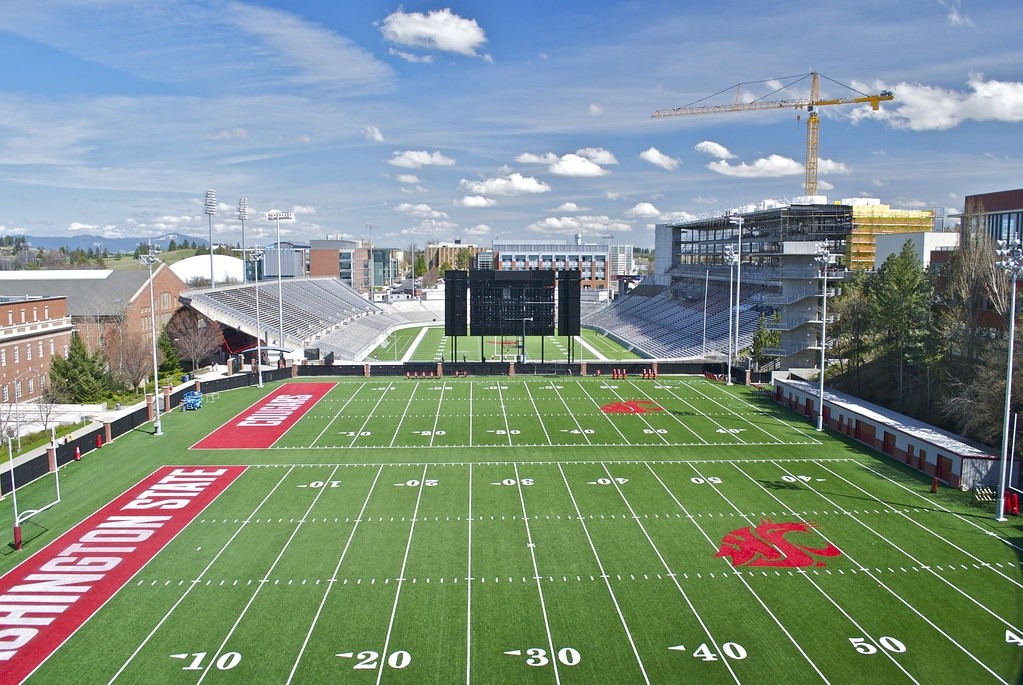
top-left (74, 445), bottom-right (81, 461)
top-left (96, 434), bottom-right (102, 448)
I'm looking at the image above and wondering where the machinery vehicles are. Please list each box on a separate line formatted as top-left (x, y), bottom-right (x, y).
top-left (182, 391), bottom-right (203, 411)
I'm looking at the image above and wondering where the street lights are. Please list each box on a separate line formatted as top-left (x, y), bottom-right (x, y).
top-left (993, 232), bottom-right (1023, 522)
top-left (148, 238), bottom-right (162, 434)
top-left (269, 211), bottom-right (291, 348)
top-left (724, 242), bottom-right (734, 383)
top-left (238, 196), bottom-right (248, 283)
top-left (813, 237), bottom-right (830, 430)
top-left (729, 216), bottom-right (744, 358)
top-left (14, 369), bottom-right (38, 448)
top-left (250, 242), bottom-right (264, 387)
top-left (205, 188), bottom-right (217, 290)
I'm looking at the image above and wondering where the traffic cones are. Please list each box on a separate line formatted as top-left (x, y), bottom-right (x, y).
top-left (1005, 491), bottom-right (1011, 513)
top-left (75, 446), bottom-right (81, 461)
top-left (96, 435), bottom-right (102, 448)
top-left (930, 477), bottom-right (939, 494)
top-left (1009, 493), bottom-right (1019, 515)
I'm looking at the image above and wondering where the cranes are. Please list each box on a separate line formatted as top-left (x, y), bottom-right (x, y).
top-left (650, 71), bottom-right (895, 195)
